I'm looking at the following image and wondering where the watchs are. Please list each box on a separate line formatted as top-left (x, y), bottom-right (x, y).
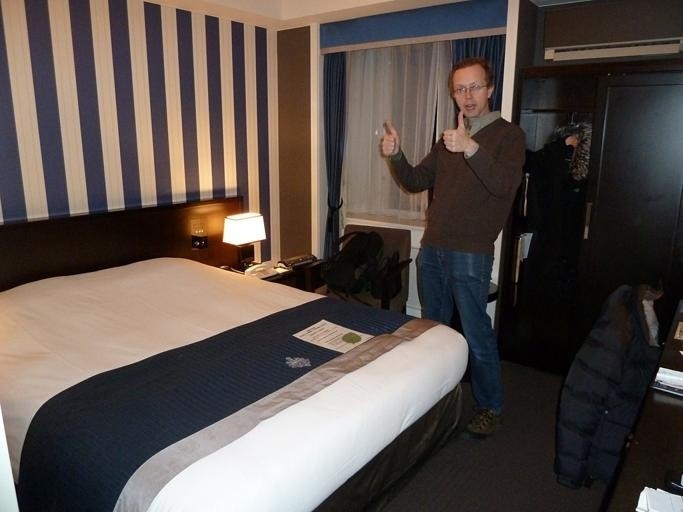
top-left (462, 143), bottom-right (481, 158)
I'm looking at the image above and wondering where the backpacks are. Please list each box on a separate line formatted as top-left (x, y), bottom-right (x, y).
top-left (324, 229), bottom-right (383, 297)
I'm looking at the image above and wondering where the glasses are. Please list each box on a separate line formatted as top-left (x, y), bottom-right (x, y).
top-left (452, 82), bottom-right (490, 94)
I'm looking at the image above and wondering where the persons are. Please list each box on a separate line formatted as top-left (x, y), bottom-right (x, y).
top-left (380, 57), bottom-right (526, 441)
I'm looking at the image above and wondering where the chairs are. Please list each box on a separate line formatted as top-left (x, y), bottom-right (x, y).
top-left (292, 225), bottom-right (411, 315)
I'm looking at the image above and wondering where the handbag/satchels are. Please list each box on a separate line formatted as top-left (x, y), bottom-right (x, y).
top-left (372, 252), bottom-right (404, 302)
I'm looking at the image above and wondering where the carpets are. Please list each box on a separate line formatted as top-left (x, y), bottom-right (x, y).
top-left (377, 357), bottom-right (607, 512)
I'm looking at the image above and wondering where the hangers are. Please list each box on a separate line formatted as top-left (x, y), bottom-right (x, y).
top-left (563, 110), bottom-right (581, 155)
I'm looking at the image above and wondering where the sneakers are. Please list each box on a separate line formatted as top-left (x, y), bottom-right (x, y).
top-left (467, 408), bottom-right (503, 435)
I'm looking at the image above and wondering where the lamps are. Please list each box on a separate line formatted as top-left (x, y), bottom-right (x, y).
top-left (221, 212), bottom-right (266, 274)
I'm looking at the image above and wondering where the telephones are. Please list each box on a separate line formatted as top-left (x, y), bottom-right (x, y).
top-left (245, 261), bottom-right (278, 279)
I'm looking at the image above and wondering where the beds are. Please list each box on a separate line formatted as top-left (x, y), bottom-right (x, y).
top-left (0, 256), bottom-right (468, 510)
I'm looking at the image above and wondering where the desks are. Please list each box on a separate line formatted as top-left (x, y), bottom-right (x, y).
top-left (448, 281), bottom-right (499, 383)
top-left (600, 297), bottom-right (683, 512)
